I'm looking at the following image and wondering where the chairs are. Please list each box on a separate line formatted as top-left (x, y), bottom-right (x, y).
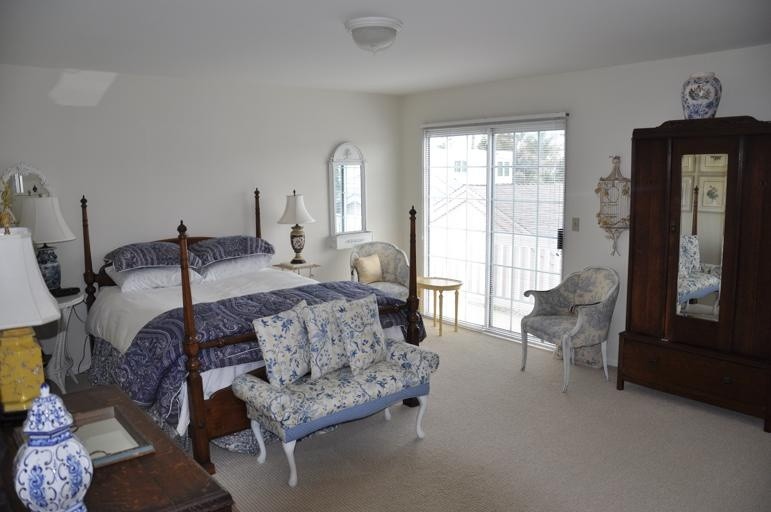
top-left (518, 263), bottom-right (620, 394)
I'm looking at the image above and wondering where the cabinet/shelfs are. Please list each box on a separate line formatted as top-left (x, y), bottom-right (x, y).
top-left (616, 113), bottom-right (770, 436)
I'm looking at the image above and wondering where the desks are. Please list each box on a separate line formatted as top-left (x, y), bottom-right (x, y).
top-left (46, 291), bottom-right (86, 395)
top-left (0, 381), bottom-right (234, 510)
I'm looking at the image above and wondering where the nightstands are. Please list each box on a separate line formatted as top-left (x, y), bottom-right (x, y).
top-left (273, 261), bottom-right (321, 278)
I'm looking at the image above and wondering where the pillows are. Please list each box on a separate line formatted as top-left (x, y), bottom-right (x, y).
top-left (199, 255), bottom-right (274, 284)
top-left (187, 234), bottom-right (275, 267)
top-left (253, 298), bottom-right (310, 390)
top-left (101, 237), bottom-right (203, 268)
top-left (329, 290), bottom-right (389, 378)
top-left (303, 293), bottom-right (350, 381)
top-left (679, 232), bottom-right (702, 274)
top-left (353, 253), bottom-right (384, 284)
top-left (104, 264), bottom-right (204, 294)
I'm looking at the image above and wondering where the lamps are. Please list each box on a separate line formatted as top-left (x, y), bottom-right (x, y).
top-left (1, 225), bottom-right (62, 413)
top-left (19, 195), bottom-right (77, 290)
top-left (276, 189), bottom-right (317, 264)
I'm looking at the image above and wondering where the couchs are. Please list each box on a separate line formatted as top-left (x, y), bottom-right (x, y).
top-left (350, 241), bottom-right (410, 302)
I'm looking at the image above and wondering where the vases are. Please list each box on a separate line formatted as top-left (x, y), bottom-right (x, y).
top-left (679, 68), bottom-right (726, 121)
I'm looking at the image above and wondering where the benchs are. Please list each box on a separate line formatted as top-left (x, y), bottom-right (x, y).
top-left (677, 262), bottom-right (721, 316)
top-left (230, 335), bottom-right (442, 488)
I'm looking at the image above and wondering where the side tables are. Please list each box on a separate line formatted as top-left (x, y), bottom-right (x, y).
top-left (416, 276), bottom-right (466, 336)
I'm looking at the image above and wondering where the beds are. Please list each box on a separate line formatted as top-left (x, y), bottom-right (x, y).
top-left (79, 185), bottom-right (426, 476)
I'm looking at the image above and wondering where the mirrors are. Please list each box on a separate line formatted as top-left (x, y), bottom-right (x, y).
top-left (675, 150), bottom-right (731, 324)
top-left (328, 140), bottom-right (374, 251)
